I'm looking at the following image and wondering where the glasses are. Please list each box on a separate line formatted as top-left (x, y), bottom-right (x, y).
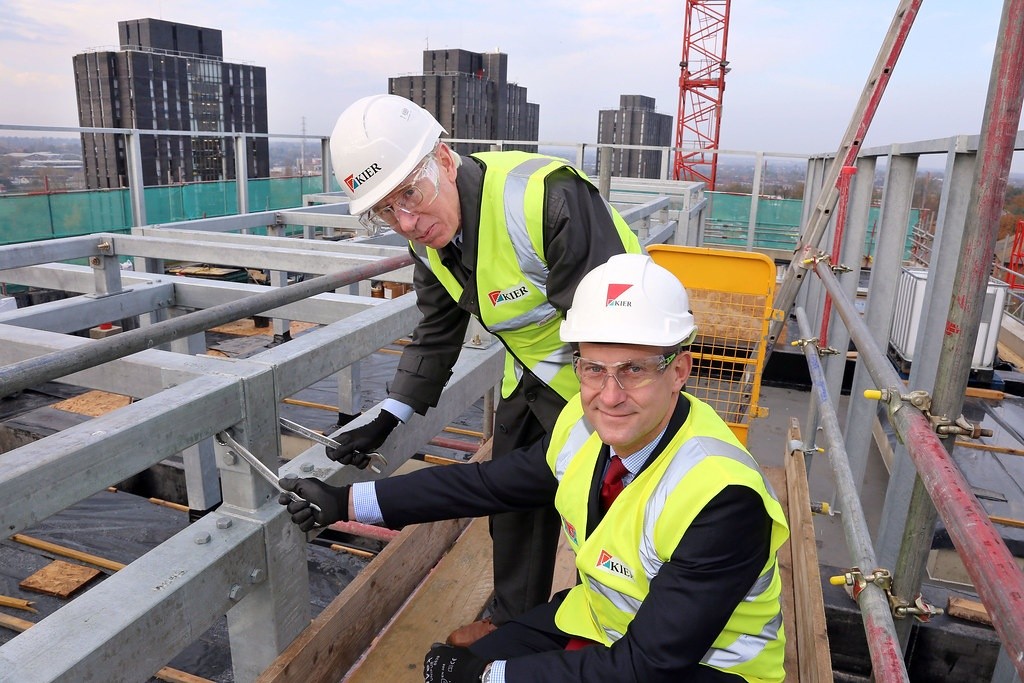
top-left (369, 155), bottom-right (430, 226)
top-left (572, 349), bottom-right (682, 391)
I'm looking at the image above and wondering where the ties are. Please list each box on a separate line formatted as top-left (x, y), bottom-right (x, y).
top-left (565, 454), bottom-right (629, 650)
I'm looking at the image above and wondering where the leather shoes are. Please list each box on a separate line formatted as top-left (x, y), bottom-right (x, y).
top-left (445, 617), bottom-right (498, 647)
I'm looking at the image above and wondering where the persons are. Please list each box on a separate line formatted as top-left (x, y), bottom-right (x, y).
top-left (326, 95), bottom-right (650, 647)
top-left (278, 255), bottom-right (791, 683)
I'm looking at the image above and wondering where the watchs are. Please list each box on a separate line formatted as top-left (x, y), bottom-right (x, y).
top-left (479, 662), bottom-right (492, 683)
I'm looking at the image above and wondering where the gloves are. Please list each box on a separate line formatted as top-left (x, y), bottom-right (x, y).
top-left (326, 410), bottom-right (398, 469)
top-left (278, 478), bottom-right (351, 534)
top-left (423, 642), bottom-right (490, 683)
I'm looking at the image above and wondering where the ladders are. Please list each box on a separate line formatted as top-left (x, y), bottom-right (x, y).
top-left (723, 0), bottom-right (924, 423)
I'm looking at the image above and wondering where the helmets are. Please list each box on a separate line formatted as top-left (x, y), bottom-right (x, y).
top-left (558, 252), bottom-right (698, 347)
top-left (330, 93), bottom-right (450, 216)
